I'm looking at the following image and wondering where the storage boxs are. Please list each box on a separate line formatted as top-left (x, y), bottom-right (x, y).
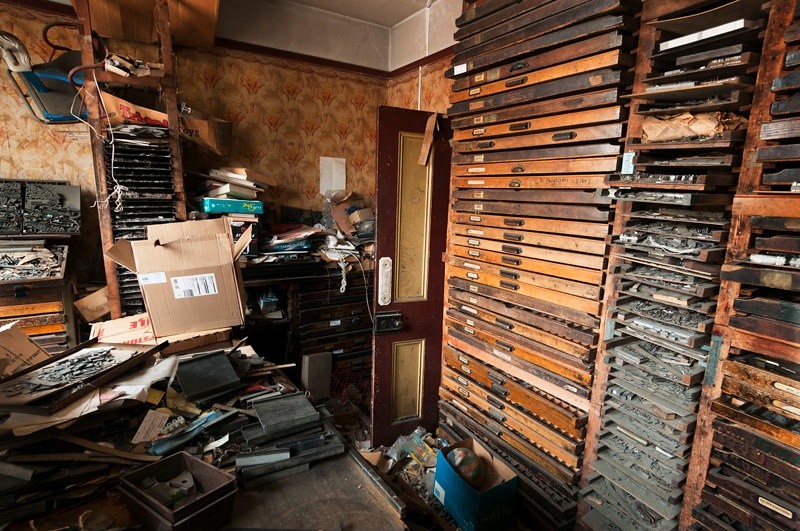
top-left (0, 320), bottom-right (53, 378)
top-left (349, 208), bottom-right (375, 225)
top-left (433, 437), bottom-right (519, 531)
top-left (117, 451), bottom-right (239, 531)
top-left (73, 184), bottom-right (311, 358)
top-left (98, 88), bottom-right (234, 158)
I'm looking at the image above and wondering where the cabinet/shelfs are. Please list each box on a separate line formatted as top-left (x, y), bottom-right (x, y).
top-left (0, 285), bottom-right (80, 357)
top-left (434, 0), bottom-right (800, 531)
top-left (76, 0), bottom-right (189, 320)
top-left (241, 264), bottom-right (373, 427)
